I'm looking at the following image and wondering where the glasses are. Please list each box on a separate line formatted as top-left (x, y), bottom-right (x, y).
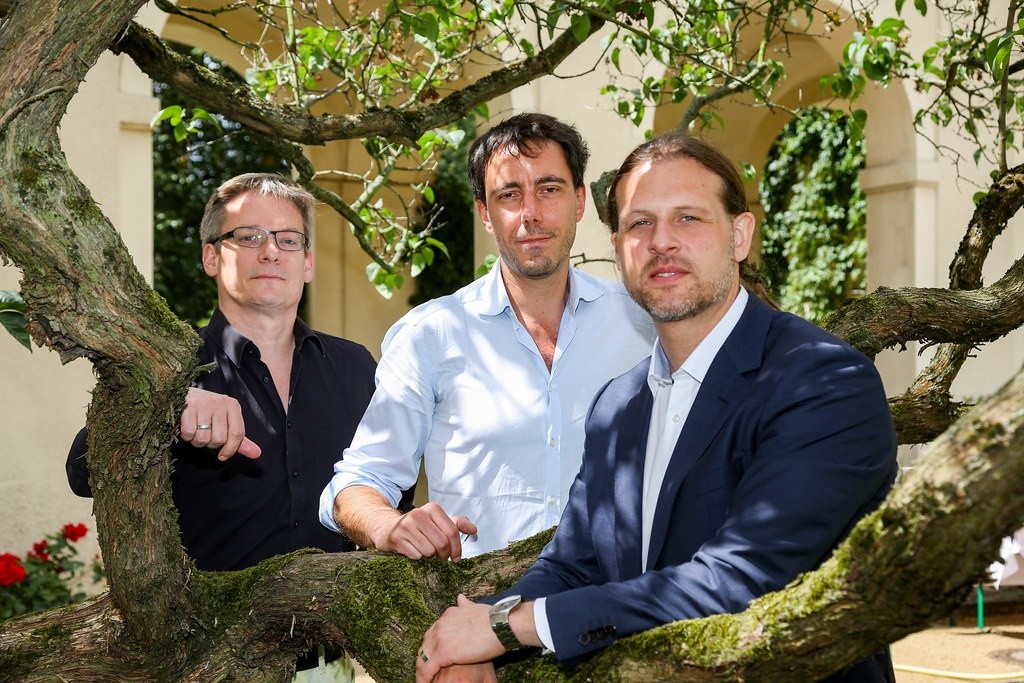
top-left (206, 227), bottom-right (310, 251)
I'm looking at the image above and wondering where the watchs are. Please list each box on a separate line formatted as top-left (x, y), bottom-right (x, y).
top-left (489, 595), bottom-right (524, 650)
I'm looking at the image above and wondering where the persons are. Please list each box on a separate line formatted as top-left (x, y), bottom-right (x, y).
top-left (319, 113), bottom-right (656, 564)
top-left (416, 134), bottom-right (899, 683)
top-left (64, 172), bottom-right (418, 683)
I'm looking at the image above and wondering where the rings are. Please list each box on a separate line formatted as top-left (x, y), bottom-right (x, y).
top-left (197, 424), bottom-right (211, 429)
top-left (420, 650), bottom-right (428, 662)
